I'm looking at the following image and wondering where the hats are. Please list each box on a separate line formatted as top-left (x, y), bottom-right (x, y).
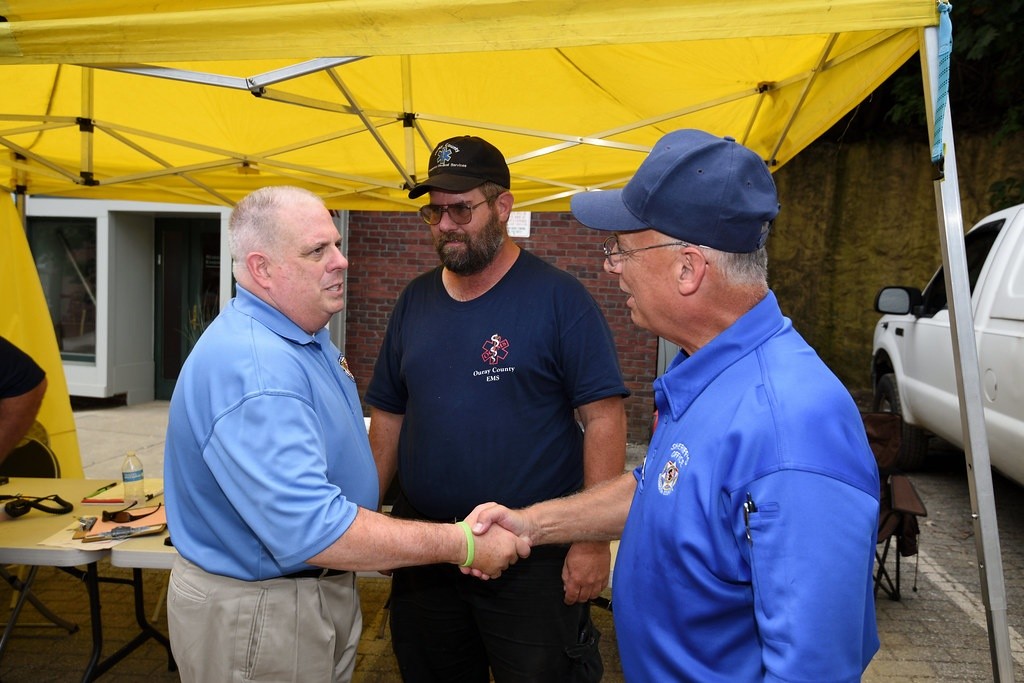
top-left (408, 135), bottom-right (510, 199)
top-left (571, 129), bottom-right (780, 253)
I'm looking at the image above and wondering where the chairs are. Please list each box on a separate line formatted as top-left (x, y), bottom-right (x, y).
top-left (859, 410), bottom-right (926, 602)
top-left (0, 438), bottom-right (79, 652)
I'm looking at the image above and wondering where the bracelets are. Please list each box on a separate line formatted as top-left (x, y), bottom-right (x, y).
top-left (456, 522), bottom-right (474, 567)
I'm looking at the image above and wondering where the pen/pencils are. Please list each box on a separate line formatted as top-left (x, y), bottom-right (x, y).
top-left (744, 488), bottom-right (757, 543)
top-left (85, 482), bottom-right (116, 500)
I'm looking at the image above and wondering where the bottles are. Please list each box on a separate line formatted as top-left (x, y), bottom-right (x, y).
top-left (121, 451), bottom-right (146, 509)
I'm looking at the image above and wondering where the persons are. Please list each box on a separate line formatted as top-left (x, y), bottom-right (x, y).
top-left (460, 130), bottom-right (880, 683)
top-left (365, 136), bottom-right (629, 683)
top-left (167, 187), bottom-right (531, 683)
top-left (0, 336), bottom-right (47, 466)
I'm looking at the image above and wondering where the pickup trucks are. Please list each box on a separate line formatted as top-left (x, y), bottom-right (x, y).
top-left (871, 203), bottom-right (1023, 484)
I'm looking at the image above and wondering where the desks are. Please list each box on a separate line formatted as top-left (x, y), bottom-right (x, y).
top-left (0, 477), bottom-right (619, 683)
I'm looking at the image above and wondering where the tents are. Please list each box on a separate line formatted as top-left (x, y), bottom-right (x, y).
top-left (0, 0), bottom-right (1013, 682)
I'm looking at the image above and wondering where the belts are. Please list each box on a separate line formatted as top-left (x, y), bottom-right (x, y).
top-left (285, 569), bottom-right (347, 579)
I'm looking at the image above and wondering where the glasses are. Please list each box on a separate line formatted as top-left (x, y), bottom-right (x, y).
top-left (102, 501), bottom-right (161, 524)
top-left (420, 193), bottom-right (501, 225)
top-left (602, 236), bottom-right (708, 267)
